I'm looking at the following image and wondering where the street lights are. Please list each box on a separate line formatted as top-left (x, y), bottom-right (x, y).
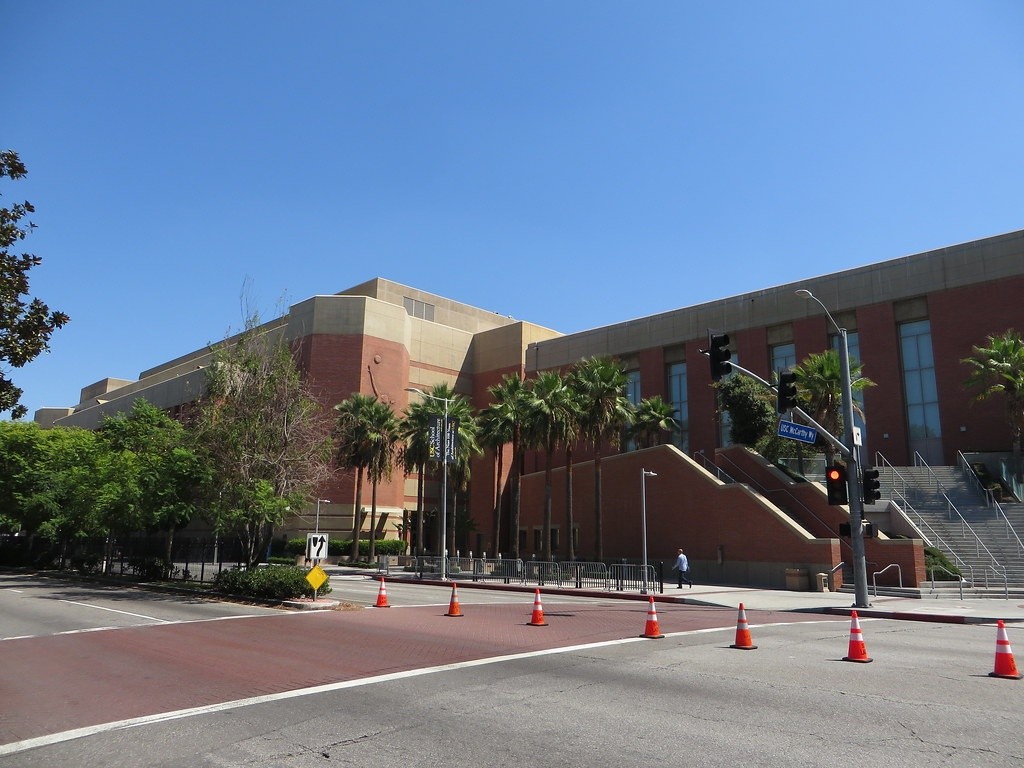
top-left (316, 498), bottom-right (330, 533)
top-left (404, 388), bottom-right (455, 580)
top-left (795, 290), bottom-right (873, 609)
top-left (641, 467), bottom-right (657, 589)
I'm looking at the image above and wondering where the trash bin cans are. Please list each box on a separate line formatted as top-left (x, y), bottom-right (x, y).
top-left (378, 555), bottom-right (389, 574)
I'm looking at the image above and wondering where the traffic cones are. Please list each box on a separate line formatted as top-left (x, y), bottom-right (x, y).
top-left (988, 620), bottom-right (1024, 680)
top-left (639, 596), bottom-right (665, 639)
top-left (372, 577), bottom-right (390, 607)
top-left (443, 583), bottom-right (465, 617)
top-left (842, 610), bottom-right (873, 664)
top-left (525, 589), bottom-right (549, 626)
top-left (729, 603), bottom-right (758, 650)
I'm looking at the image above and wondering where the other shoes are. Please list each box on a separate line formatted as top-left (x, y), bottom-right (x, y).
top-left (677, 586), bottom-right (682, 588)
top-left (689, 581), bottom-right (692, 588)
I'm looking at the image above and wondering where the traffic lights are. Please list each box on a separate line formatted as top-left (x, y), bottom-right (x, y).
top-left (777, 367), bottom-right (797, 414)
top-left (839, 523), bottom-right (850, 536)
top-left (862, 467), bottom-right (881, 505)
top-left (825, 465), bottom-right (847, 505)
top-left (708, 328), bottom-right (732, 382)
top-left (866, 524), bottom-right (878, 537)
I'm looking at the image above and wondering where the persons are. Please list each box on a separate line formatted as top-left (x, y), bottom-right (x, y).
top-left (672, 549), bottom-right (692, 589)
